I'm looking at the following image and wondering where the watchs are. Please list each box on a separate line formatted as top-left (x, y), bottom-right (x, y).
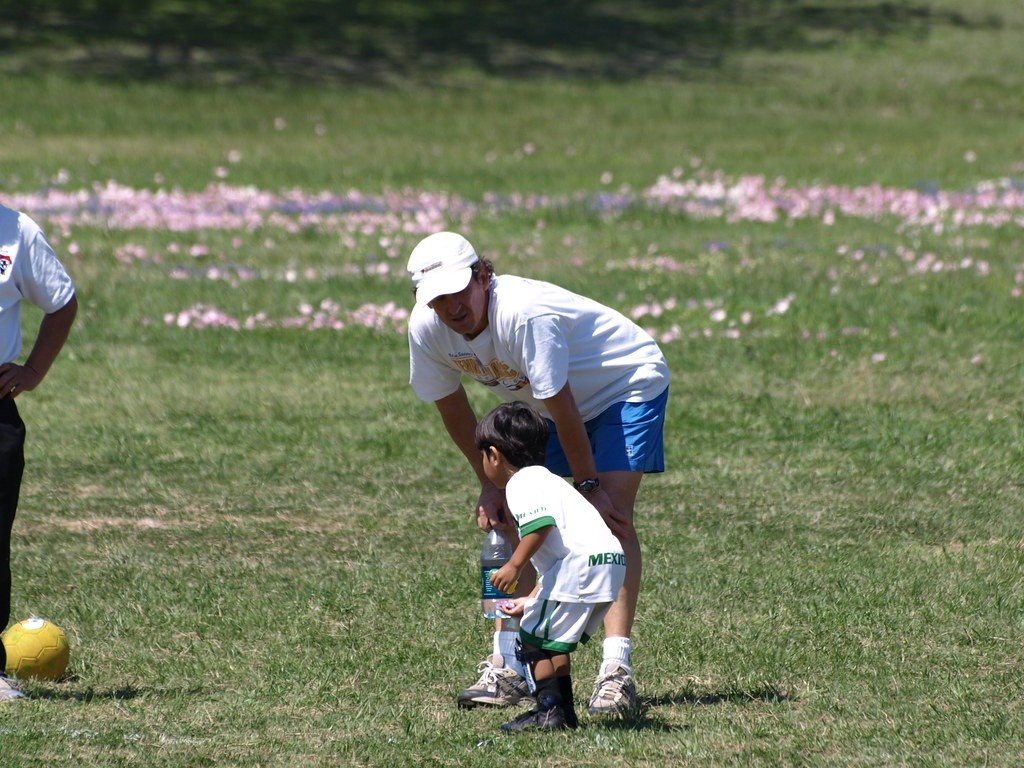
top-left (573, 477), bottom-right (600, 492)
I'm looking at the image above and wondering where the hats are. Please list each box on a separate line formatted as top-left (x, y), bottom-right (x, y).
top-left (406, 232), bottom-right (479, 308)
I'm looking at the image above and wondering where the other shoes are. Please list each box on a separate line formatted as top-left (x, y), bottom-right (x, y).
top-left (0, 678), bottom-right (26, 704)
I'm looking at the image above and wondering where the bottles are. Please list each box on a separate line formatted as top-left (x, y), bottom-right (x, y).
top-left (480, 520), bottom-right (515, 619)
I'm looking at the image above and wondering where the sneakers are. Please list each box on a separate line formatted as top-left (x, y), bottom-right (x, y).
top-left (588, 663), bottom-right (633, 719)
top-left (457, 654), bottom-right (531, 711)
top-left (501, 704), bottom-right (579, 734)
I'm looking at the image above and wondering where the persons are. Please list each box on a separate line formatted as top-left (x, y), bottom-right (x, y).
top-left (0, 204), bottom-right (79, 704)
top-left (475, 400), bottom-right (628, 734)
top-left (407, 231), bottom-right (671, 716)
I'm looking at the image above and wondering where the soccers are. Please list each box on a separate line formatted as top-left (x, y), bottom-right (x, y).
top-left (3, 618), bottom-right (70, 681)
